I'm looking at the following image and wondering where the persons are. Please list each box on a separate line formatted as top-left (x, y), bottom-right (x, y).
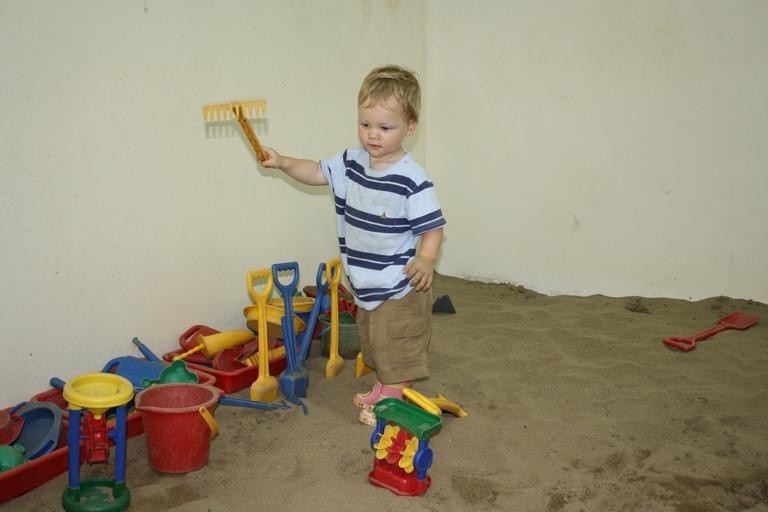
top-left (257, 65), bottom-right (446, 426)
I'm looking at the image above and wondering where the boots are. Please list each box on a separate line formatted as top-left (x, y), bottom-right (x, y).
top-left (359, 380), bottom-right (414, 426)
top-left (352, 381), bottom-right (382, 408)
